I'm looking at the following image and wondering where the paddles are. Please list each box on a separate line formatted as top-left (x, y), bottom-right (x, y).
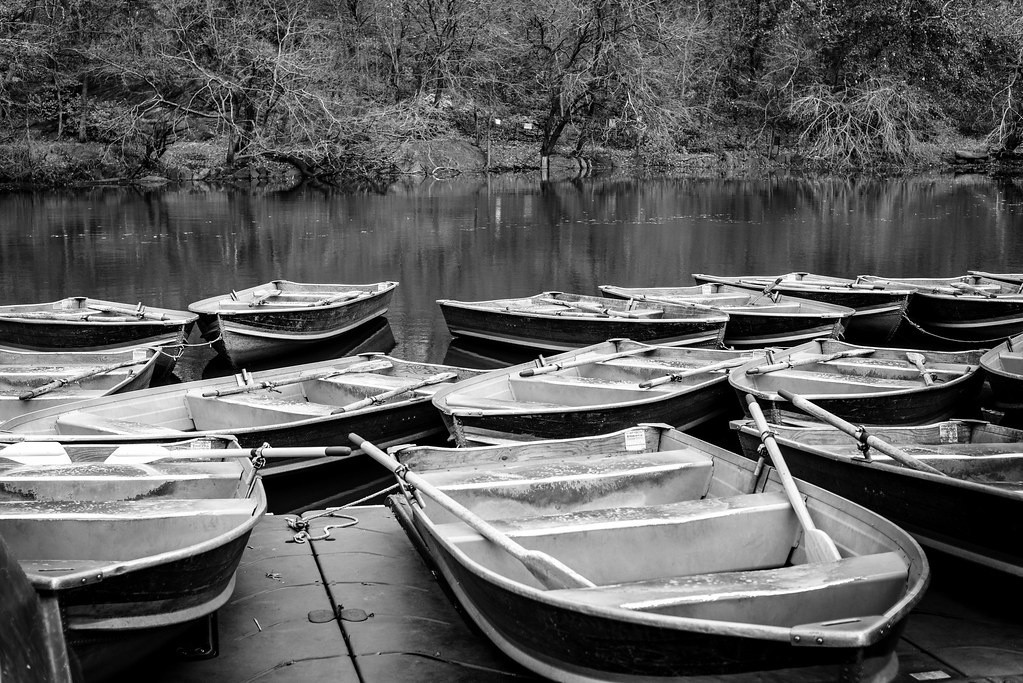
top-left (741, 277), bottom-right (997, 307)
top-left (201, 360), bottom-right (393, 398)
top-left (18, 356), bottom-right (150, 401)
top-left (330, 372), bottom-right (459, 417)
top-left (348, 434), bottom-right (598, 592)
top-left (903, 351), bottom-right (936, 386)
top-left (0, 304), bottom-right (171, 322)
top-left (778, 390), bottom-right (948, 477)
top-left (637, 347), bottom-right (876, 387)
top-left (520, 344), bottom-right (658, 379)
top-left (0, 440), bottom-right (351, 464)
top-left (248, 288), bottom-right (282, 308)
top-left (307, 289), bottom-right (362, 305)
top-left (744, 391), bottom-right (842, 567)
top-left (503, 296), bottom-right (638, 320)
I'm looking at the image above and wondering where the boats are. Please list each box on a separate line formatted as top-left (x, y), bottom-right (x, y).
top-left (0, 434), bottom-right (272, 683)
top-left (729, 415), bottom-right (1022, 600)
top-left (384, 419), bottom-right (931, 683)
top-left (0, 271), bottom-right (1023, 515)
